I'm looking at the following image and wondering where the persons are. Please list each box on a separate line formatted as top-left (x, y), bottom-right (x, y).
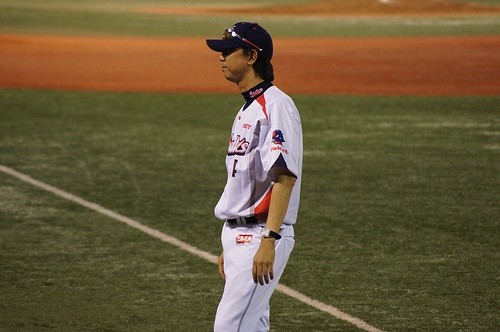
top-left (206, 21), bottom-right (304, 332)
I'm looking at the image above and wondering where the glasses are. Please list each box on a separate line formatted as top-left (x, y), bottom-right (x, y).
top-left (223, 27), bottom-right (263, 51)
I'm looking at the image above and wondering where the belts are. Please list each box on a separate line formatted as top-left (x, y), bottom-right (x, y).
top-left (226, 216), bottom-right (259, 226)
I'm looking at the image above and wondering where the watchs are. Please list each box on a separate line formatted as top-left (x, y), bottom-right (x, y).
top-left (260, 228), bottom-right (283, 241)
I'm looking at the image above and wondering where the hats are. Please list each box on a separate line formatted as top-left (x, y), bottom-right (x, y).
top-left (206, 22), bottom-right (273, 60)
